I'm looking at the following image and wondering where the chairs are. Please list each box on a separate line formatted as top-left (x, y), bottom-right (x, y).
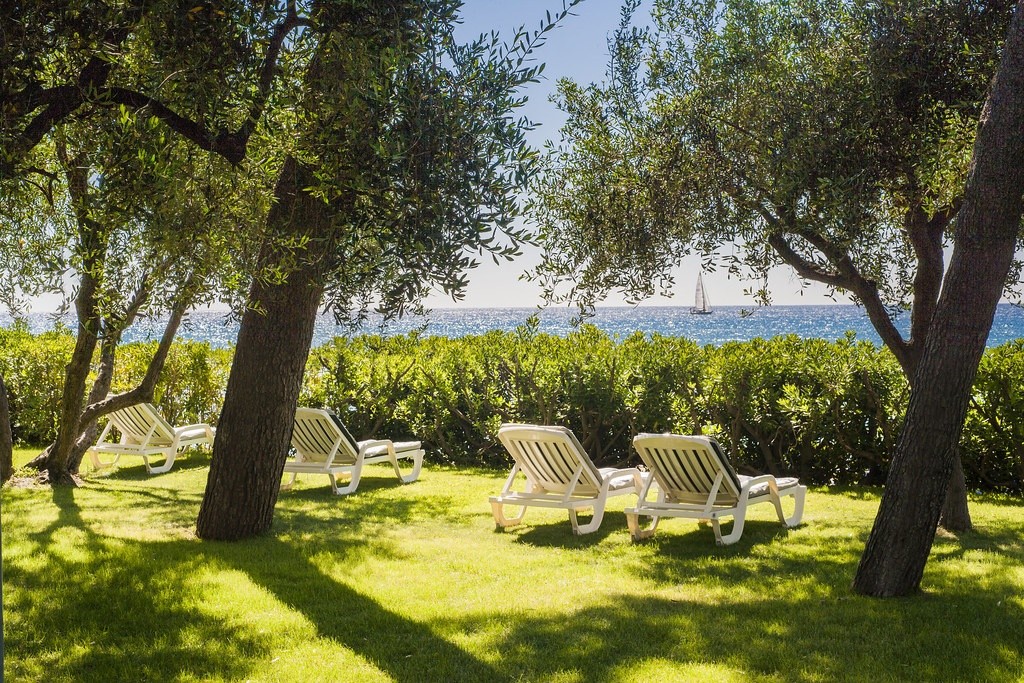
top-left (623, 433), bottom-right (808, 545)
top-left (488, 423), bottom-right (658, 535)
top-left (86, 393), bottom-right (216, 474)
top-left (283, 408), bottom-right (425, 494)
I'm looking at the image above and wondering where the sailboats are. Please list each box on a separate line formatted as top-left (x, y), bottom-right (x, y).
top-left (689, 270), bottom-right (714, 314)
